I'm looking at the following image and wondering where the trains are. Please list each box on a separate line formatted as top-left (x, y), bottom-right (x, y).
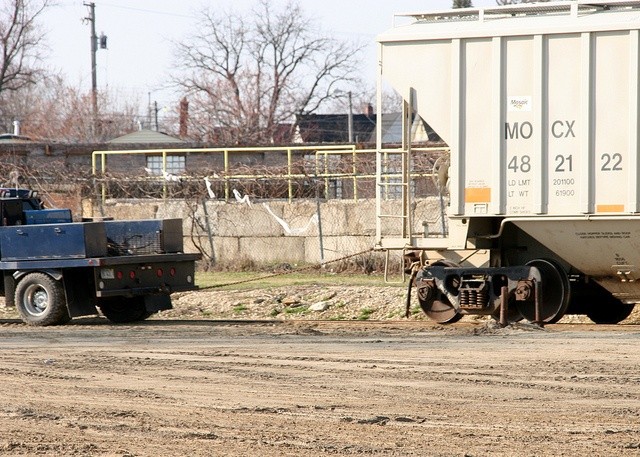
top-left (374, 0), bottom-right (640, 326)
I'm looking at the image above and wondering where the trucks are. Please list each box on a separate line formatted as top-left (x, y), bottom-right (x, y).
top-left (0, 187), bottom-right (201, 326)
